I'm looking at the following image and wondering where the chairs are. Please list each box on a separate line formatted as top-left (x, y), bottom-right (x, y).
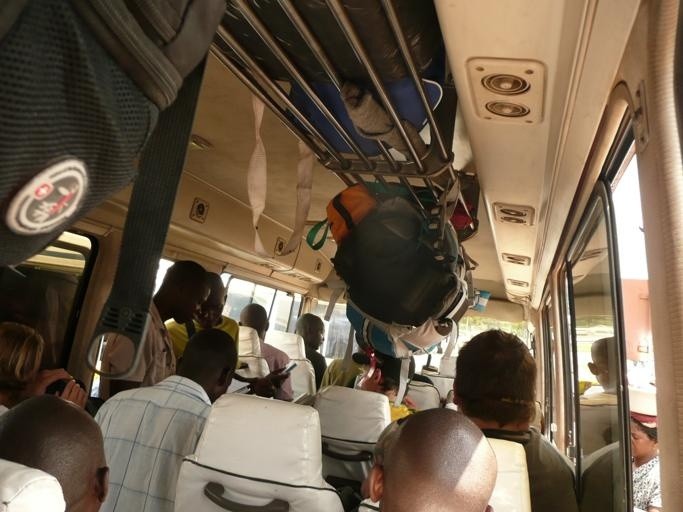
top-left (228, 327), bottom-right (268, 391)
top-left (438, 356), bottom-right (457, 399)
top-left (579, 442), bottom-right (616, 511)
top-left (173, 392), bottom-right (346, 512)
top-left (313, 386), bottom-right (390, 494)
top-left (487, 438), bottom-right (531, 512)
top-left (580, 396), bottom-right (619, 452)
top-left (0, 457), bottom-right (67, 510)
top-left (404, 381), bottom-right (440, 412)
top-left (264, 330), bottom-right (316, 403)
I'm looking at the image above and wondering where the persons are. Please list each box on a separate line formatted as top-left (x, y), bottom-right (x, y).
top-left (0, 317), bottom-right (88, 413)
top-left (628, 384), bottom-right (662, 511)
top-left (238, 303), bottom-right (294, 402)
top-left (587, 337), bottom-right (617, 394)
top-left (165, 271), bottom-right (291, 399)
top-left (295, 313), bottom-right (327, 390)
top-left (94, 328), bottom-right (239, 510)
top-left (320, 357), bottom-right (368, 390)
top-left (1, 394), bottom-right (110, 511)
top-left (359, 327), bottom-right (580, 511)
top-left (98, 261), bottom-right (211, 402)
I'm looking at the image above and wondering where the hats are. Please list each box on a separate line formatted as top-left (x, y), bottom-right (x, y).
top-left (352, 350), bottom-right (414, 384)
top-left (628, 386), bottom-right (656, 428)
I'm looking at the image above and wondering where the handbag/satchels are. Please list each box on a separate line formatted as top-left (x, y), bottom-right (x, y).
top-left (0, 0), bottom-right (228, 266)
